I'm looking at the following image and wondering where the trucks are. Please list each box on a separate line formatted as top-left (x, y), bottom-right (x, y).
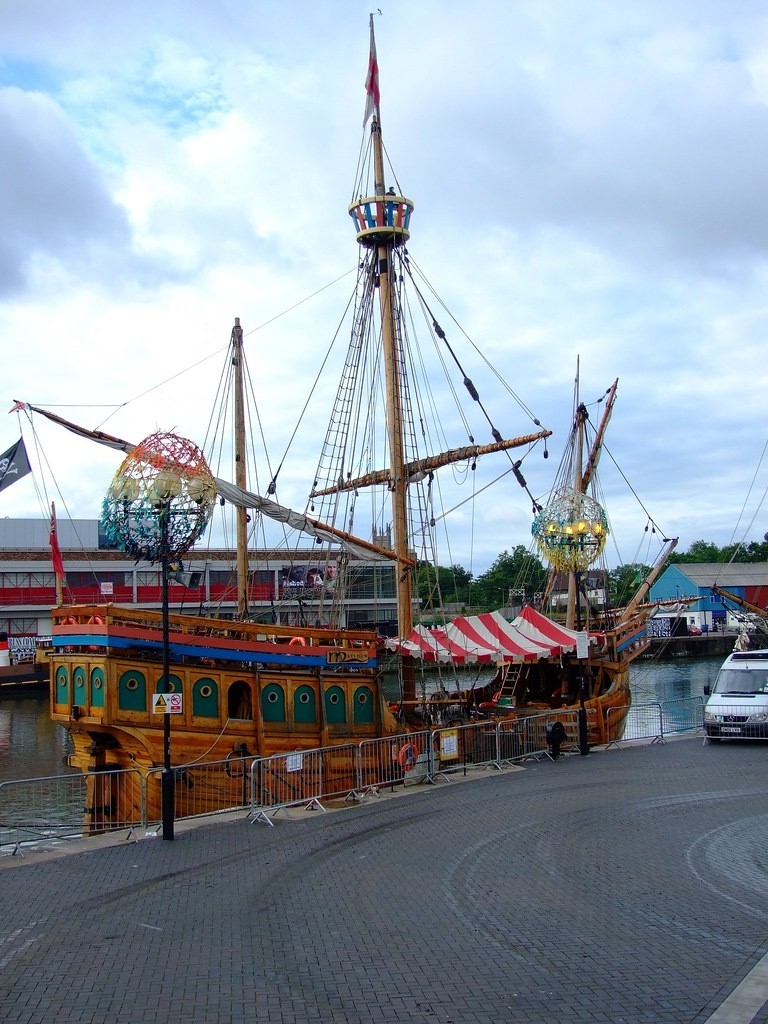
top-left (730, 614), bottom-right (760, 632)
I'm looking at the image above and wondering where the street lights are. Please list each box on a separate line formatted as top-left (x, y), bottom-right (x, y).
top-left (498, 588), bottom-right (504, 608)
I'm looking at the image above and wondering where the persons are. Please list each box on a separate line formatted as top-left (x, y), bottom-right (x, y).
top-left (307, 568), bottom-right (324, 590)
top-left (546, 722), bottom-right (568, 761)
top-left (324, 565), bottom-right (337, 581)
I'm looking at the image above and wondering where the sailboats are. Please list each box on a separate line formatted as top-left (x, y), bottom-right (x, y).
top-left (10, 12), bottom-right (707, 835)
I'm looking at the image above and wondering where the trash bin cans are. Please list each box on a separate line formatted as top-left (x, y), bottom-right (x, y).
top-left (718, 625), bottom-right (722, 631)
top-left (712, 625), bottom-right (717, 632)
top-left (702, 625), bottom-right (708, 632)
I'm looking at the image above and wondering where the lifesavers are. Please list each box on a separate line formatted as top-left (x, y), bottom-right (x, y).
top-left (60, 618), bottom-right (78, 652)
top-left (85, 616), bottom-right (104, 651)
top-left (398, 743), bottom-right (419, 771)
top-left (286, 636), bottom-right (307, 657)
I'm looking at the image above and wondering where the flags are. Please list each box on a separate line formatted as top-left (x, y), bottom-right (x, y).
top-left (0, 437), bottom-right (31, 492)
top-left (49, 510), bottom-right (65, 579)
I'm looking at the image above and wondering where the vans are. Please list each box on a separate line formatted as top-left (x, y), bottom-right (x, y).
top-left (702, 649), bottom-right (768, 743)
top-left (748, 613), bottom-right (760, 621)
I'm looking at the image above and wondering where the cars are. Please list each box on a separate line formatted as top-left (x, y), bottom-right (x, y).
top-left (686, 624), bottom-right (703, 636)
